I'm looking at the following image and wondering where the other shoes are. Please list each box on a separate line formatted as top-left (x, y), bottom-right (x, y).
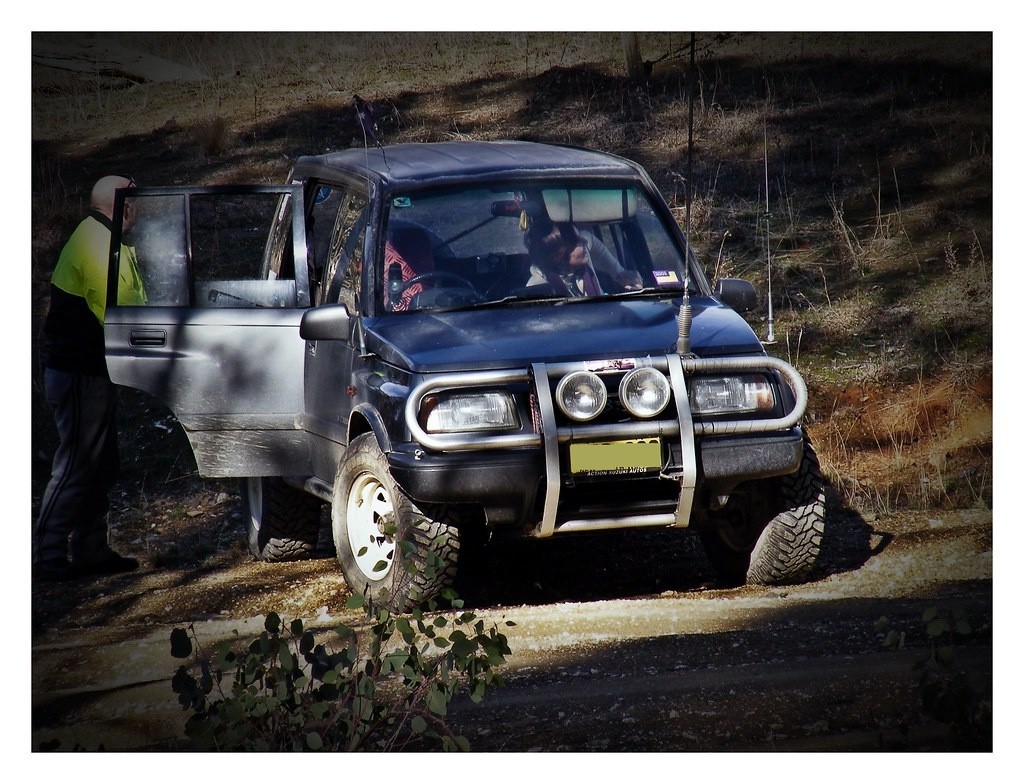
top-left (33, 548), bottom-right (140, 582)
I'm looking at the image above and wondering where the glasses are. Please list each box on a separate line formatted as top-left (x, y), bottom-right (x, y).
top-left (126, 176), bottom-right (136, 188)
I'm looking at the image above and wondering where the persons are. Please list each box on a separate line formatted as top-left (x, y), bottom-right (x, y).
top-left (31, 176), bottom-right (150, 601)
top-left (525, 215), bottom-right (643, 297)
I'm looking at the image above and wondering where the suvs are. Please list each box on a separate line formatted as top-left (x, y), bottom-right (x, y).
top-left (104, 141), bottom-right (826, 616)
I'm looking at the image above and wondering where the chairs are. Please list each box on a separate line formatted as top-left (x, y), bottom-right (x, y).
top-left (358, 227), bottom-right (435, 312)
